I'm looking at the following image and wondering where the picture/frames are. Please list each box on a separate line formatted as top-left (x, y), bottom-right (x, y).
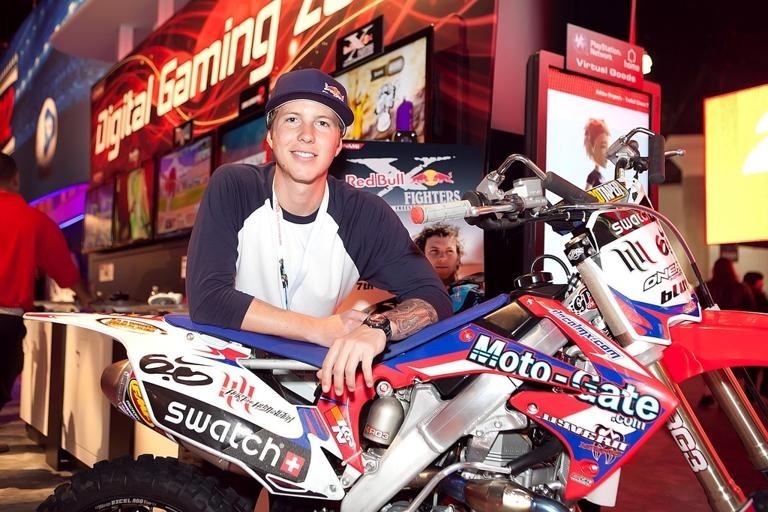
top-left (214, 102), bottom-right (270, 177)
top-left (111, 155), bottom-right (159, 249)
top-left (336, 13), bottom-right (385, 76)
top-left (171, 119), bottom-right (195, 152)
top-left (324, 23), bottom-right (436, 149)
top-left (82, 175), bottom-right (115, 254)
top-left (151, 127), bottom-right (218, 244)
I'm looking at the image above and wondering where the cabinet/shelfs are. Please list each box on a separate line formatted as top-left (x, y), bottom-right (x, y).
top-left (8, 306), bottom-right (194, 471)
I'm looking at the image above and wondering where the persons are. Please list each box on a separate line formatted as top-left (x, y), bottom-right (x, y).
top-left (0, 154), bottom-right (89, 455)
top-left (692, 256), bottom-right (765, 406)
top-left (414, 221), bottom-right (466, 291)
top-left (192, 67), bottom-right (454, 512)
top-left (581, 118), bottom-right (612, 189)
top-left (742, 270), bottom-right (767, 398)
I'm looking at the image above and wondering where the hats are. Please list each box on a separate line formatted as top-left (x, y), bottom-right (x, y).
top-left (265, 68), bottom-right (354, 139)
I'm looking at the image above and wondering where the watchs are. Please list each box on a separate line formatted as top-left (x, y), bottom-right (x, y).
top-left (362, 312), bottom-right (393, 342)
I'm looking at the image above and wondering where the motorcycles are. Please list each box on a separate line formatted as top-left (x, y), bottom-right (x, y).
top-left (23, 127), bottom-right (768, 512)
